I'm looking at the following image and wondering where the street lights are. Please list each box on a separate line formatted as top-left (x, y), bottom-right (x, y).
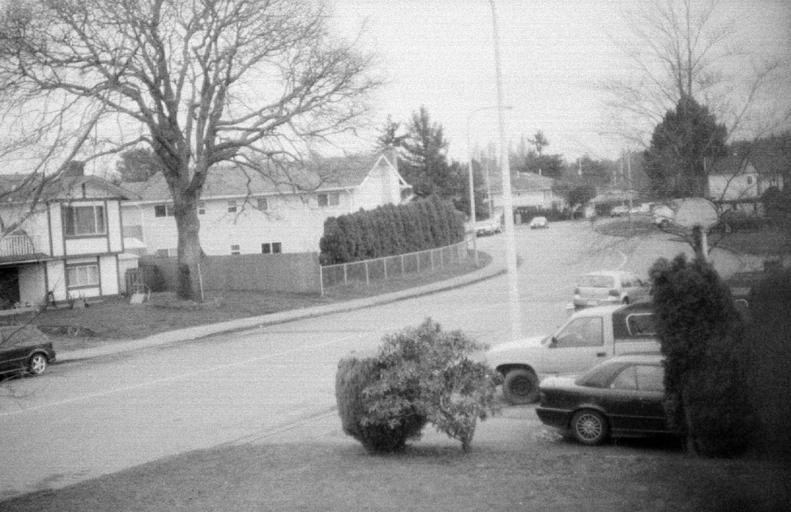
top-left (466, 100), bottom-right (517, 267)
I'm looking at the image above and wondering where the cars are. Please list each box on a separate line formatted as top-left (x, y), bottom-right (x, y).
top-left (529, 215), bottom-right (548, 229)
top-left (476, 216), bottom-right (501, 236)
top-left (607, 201), bottom-right (673, 231)
top-left (0, 323), bottom-right (59, 381)
